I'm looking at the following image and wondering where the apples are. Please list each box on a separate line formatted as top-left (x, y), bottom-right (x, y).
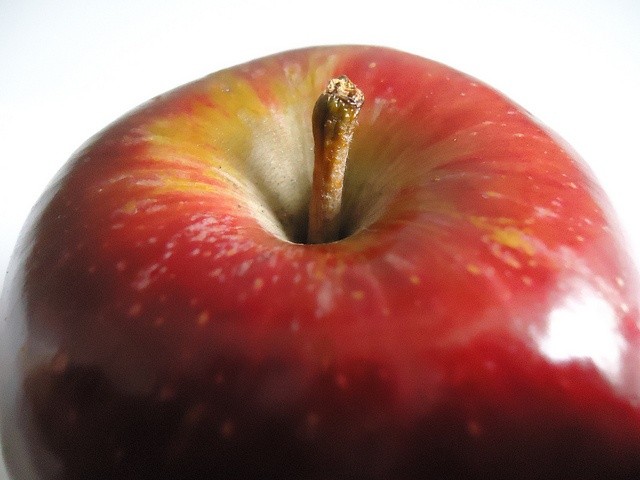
top-left (0, 43), bottom-right (639, 476)
